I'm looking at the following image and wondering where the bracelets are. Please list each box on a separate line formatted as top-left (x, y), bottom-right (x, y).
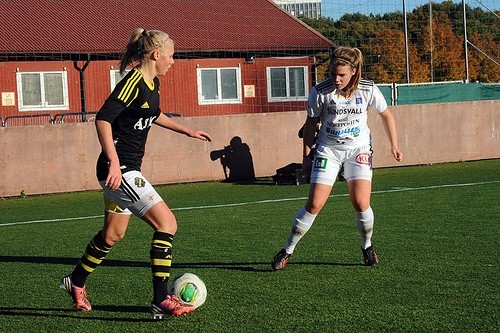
top-left (303, 155), bottom-right (311, 158)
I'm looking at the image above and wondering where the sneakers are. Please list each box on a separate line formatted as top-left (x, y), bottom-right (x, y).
top-left (60, 274), bottom-right (91, 310)
top-left (361, 245), bottom-right (379, 265)
top-left (150, 295), bottom-right (196, 319)
top-left (271, 248), bottom-right (291, 270)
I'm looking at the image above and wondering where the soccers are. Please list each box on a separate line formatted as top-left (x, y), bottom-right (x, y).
top-left (170, 272), bottom-right (207, 309)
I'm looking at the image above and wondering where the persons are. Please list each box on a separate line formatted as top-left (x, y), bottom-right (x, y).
top-left (271, 46), bottom-right (403, 270)
top-left (58, 27), bottom-right (212, 318)
